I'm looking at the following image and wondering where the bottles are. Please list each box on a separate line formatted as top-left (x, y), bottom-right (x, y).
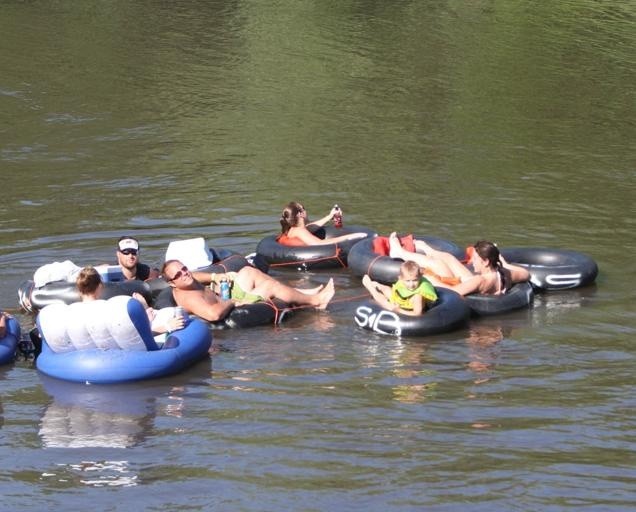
top-left (118, 236), bottom-right (139, 252)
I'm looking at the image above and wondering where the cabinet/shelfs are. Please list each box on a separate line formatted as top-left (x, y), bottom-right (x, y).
top-left (334, 205), bottom-right (343, 229)
top-left (219, 279), bottom-right (230, 300)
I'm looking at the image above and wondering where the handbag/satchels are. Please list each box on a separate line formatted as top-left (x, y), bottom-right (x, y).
top-left (164, 323), bottom-right (172, 333)
top-left (211, 272), bottom-right (216, 282)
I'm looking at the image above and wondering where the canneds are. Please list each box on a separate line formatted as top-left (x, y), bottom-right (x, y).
top-left (16, 246), bottom-right (293, 383)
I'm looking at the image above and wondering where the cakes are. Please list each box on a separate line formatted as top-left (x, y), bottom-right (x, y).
top-left (220, 282), bottom-right (230, 300)
top-left (174, 307), bottom-right (183, 319)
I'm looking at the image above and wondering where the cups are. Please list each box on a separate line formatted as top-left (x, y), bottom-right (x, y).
top-left (118, 249), bottom-right (137, 255)
top-left (169, 266), bottom-right (188, 282)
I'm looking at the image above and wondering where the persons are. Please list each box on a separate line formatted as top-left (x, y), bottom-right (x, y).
top-left (0, 311), bottom-right (10, 338)
top-left (163, 260), bottom-right (335, 321)
top-left (362, 261), bottom-right (439, 316)
top-left (75, 266), bottom-right (186, 336)
top-left (102, 237), bottom-right (160, 282)
top-left (389, 231), bottom-right (531, 296)
top-left (279, 202), bottom-right (368, 246)
